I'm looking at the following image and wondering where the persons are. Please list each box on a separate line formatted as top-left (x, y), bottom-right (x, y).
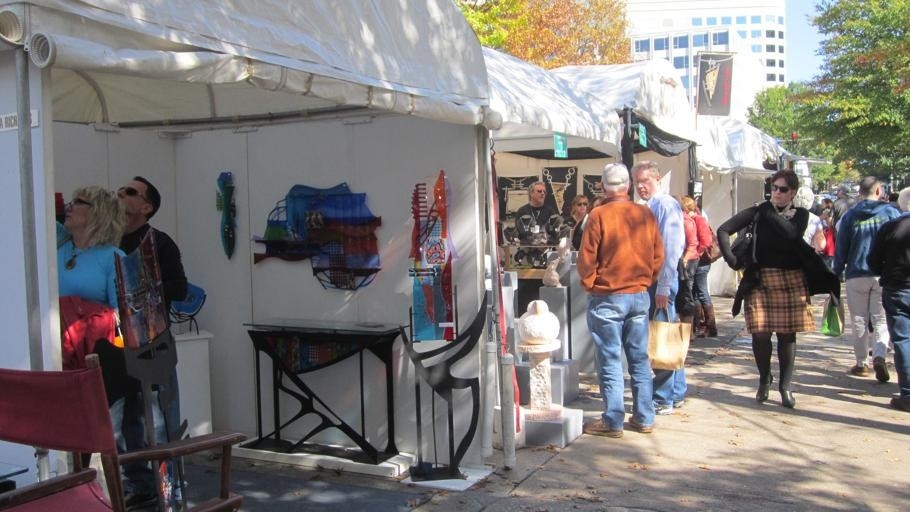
top-left (514, 175), bottom-right (716, 349)
top-left (630, 160), bottom-right (692, 413)
top-left (717, 168), bottom-right (840, 408)
top-left (866, 185), bottom-right (910, 414)
top-left (575, 163), bottom-right (665, 438)
top-left (116, 176), bottom-right (187, 504)
top-left (56, 185), bottom-right (159, 507)
top-left (831, 175), bottom-right (903, 381)
top-left (794, 175), bottom-right (903, 282)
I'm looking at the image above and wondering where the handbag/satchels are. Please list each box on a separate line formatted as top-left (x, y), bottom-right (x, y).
top-left (730, 223), bottom-right (753, 255)
top-left (699, 232), bottom-right (722, 266)
top-left (820, 296), bottom-right (846, 336)
top-left (822, 228), bottom-right (836, 257)
top-left (648, 321), bottom-right (692, 370)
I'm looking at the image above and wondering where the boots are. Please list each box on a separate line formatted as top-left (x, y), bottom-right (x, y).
top-left (680, 304), bottom-right (717, 341)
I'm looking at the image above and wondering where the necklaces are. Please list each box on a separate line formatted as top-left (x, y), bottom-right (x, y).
top-left (65, 239), bottom-right (89, 271)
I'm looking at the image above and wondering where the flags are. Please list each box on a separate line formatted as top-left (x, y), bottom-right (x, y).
top-left (696, 51), bottom-right (733, 117)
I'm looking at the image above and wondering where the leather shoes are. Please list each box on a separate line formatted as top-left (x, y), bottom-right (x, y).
top-left (781, 391), bottom-right (795, 407)
top-left (756, 375), bottom-right (772, 402)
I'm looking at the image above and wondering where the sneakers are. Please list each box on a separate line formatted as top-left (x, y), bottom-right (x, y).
top-left (851, 365), bottom-right (869, 377)
top-left (891, 397), bottom-right (910, 411)
top-left (873, 357), bottom-right (889, 382)
top-left (629, 418), bottom-right (652, 433)
top-left (583, 419), bottom-right (624, 437)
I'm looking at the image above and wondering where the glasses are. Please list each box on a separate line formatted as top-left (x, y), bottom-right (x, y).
top-left (771, 185), bottom-right (788, 193)
top-left (119, 188), bottom-right (147, 202)
top-left (69, 198), bottom-right (91, 207)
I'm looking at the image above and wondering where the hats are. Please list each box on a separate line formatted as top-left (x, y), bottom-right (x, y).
top-left (603, 163), bottom-right (629, 185)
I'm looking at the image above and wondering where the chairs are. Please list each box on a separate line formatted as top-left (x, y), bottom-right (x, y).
top-left (1, 355), bottom-right (247, 512)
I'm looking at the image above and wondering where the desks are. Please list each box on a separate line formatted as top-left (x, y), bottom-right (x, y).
top-left (166, 327), bottom-right (217, 439)
top-left (242, 322), bottom-right (409, 464)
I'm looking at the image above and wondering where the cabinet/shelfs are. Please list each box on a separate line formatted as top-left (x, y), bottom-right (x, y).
top-left (502, 239), bottom-right (566, 282)
